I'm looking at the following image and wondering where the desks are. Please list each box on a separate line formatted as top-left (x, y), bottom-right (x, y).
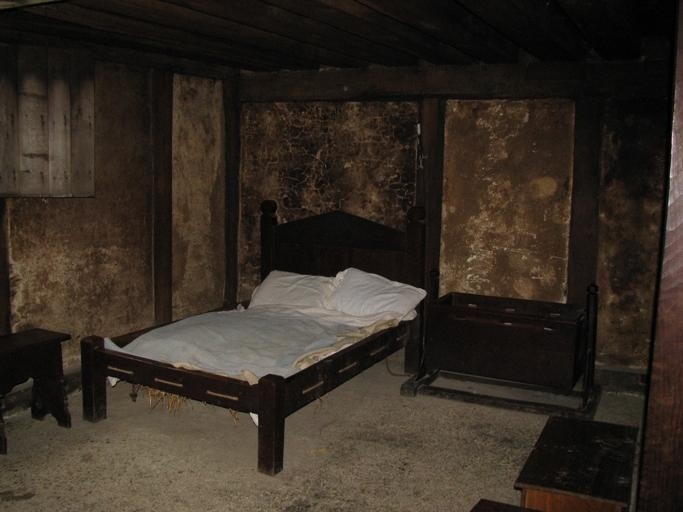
top-left (517, 412), bottom-right (638, 512)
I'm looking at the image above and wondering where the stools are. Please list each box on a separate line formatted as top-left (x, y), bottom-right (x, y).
top-left (0, 328), bottom-right (73, 454)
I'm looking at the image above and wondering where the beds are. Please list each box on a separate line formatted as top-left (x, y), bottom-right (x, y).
top-left (401, 285), bottom-right (606, 412)
top-left (80, 199), bottom-right (423, 476)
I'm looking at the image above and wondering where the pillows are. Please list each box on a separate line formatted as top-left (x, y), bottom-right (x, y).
top-left (324, 267), bottom-right (428, 325)
top-left (249, 269), bottom-right (336, 318)
